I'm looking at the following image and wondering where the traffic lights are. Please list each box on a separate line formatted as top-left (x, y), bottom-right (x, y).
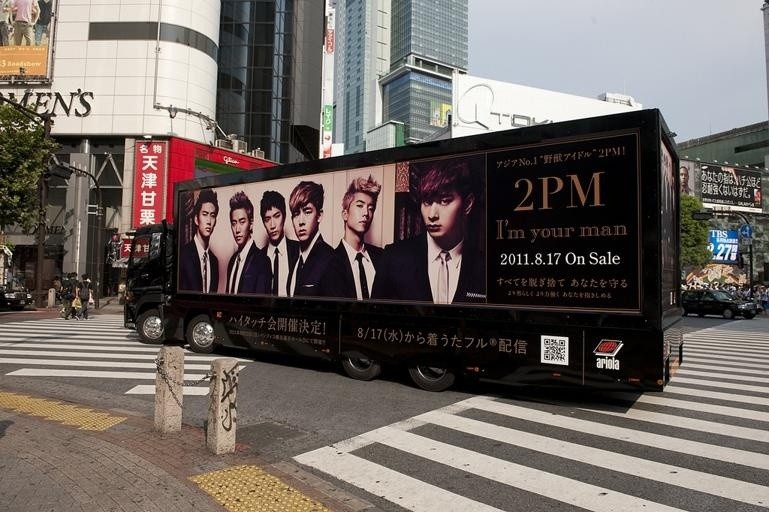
top-left (691, 212), bottom-right (713, 220)
top-left (736, 256), bottom-right (744, 269)
top-left (47, 164), bottom-right (73, 180)
top-left (111, 233), bottom-right (120, 243)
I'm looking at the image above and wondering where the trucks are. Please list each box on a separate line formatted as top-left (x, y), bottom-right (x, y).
top-left (124, 107), bottom-right (684, 393)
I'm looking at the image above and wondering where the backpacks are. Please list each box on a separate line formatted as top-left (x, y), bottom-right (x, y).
top-left (80, 282), bottom-right (90, 300)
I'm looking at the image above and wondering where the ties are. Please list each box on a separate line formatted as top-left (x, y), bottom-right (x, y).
top-left (356, 253), bottom-right (369, 299)
top-left (203, 252), bottom-right (208, 292)
top-left (438, 252), bottom-right (449, 304)
top-left (231, 254), bottom-right (241, 294)
top-left (273, 248), bottom-right (279, 296)
top-left (294, 255), bottom-right (303, 295)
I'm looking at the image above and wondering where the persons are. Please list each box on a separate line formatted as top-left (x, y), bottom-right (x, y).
top-left (260, 190), bottom-right (299, 295)
top-left (685, 277), bottom-right (769, 318)
top-left (179, 190), bottom-right (221, 294)
top-left (286, 181), bottom-right (333, 296)
top-left (678, 166), bottom-right (694, 195)
top-left (224, 189), bottom-right (270, 293)
top-left (51, 270), bottom-right (94, 321)
top-left (386, 167), bottom-right (486, 304)
top-left (325, 175), bottom-right (384, 299)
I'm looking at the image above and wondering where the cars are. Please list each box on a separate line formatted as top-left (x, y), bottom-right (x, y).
top-left (681, 289), bottom-right (758, 320)
top-left (0, 284), bottom-right (32, 311)
